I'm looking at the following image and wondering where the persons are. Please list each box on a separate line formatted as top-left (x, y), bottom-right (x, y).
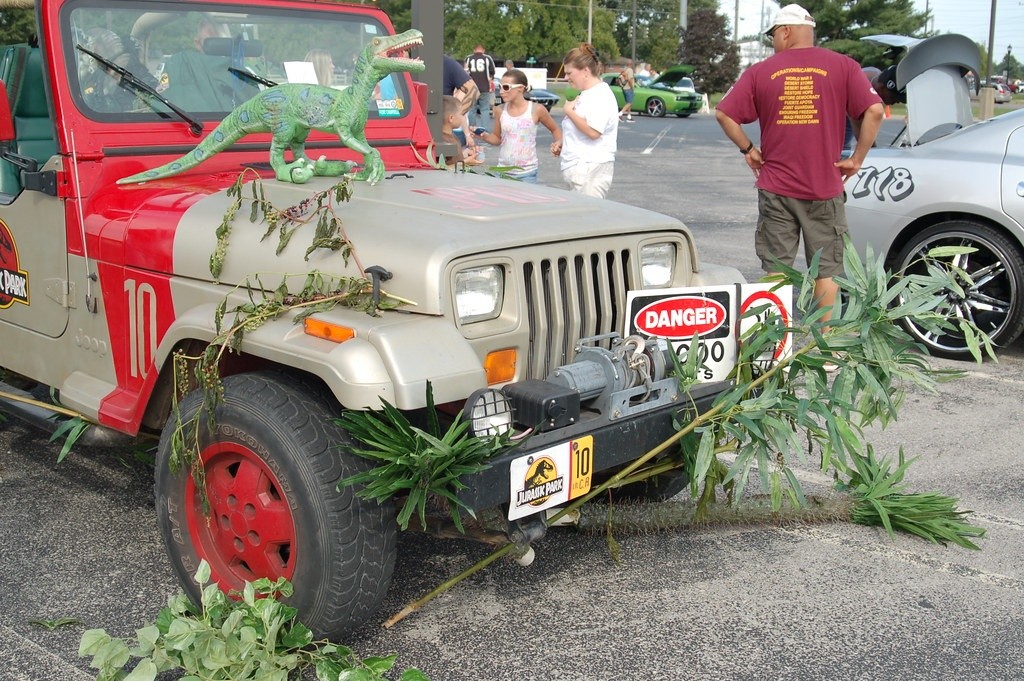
top-left (194, 17), bottom-right (226, 55)
top-left (715, 4), bottom-right (885, 373)
top-left (640, 63), bottom-right (652, 76)
top-left (303, 49), bottom-right (335, 89)
top-left (505, 59), bottom-right (515, 71)
top-left (469, 69), bottom-right (563, 184)
top-left (442, 54), bottom-right (479, 172)
top-left (552, 43), bottom-right (619, 199)
top-left (465, 43), bottom-right (495, 131)
top-left (649, 68), bottom-right (659, 82)
top-left (618, 62), bottom-right (636, 123)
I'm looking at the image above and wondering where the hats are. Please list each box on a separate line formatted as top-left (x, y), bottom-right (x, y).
top-left (760, 3), bottom-right (816, 34)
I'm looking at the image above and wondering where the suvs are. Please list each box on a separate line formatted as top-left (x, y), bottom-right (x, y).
top-left (1, 0), bottom-right (799, 653)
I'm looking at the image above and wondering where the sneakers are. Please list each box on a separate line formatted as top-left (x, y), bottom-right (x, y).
top-left (618, 113), bottom-right (624, 122)
top-left (626, 119), bottom-right (635, 122)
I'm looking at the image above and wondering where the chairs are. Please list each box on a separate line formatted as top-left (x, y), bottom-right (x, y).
top-left (0, 46), bottom-right (58, 197)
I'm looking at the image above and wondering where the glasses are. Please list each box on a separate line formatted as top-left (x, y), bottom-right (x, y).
top-left (499, 83), bottom-right (524, 92)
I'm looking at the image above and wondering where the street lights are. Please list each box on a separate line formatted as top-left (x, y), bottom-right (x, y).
top-left (1006, 44), bottom-right (1012, 84)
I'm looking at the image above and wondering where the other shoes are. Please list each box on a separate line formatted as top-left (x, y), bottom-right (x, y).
top-left (822, 352), bottom-right (843, 371)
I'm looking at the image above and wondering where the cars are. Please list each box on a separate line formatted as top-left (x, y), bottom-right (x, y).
top-left (993, 83), bottom-right (1013, 102)
top-left (979, 74), bottom-right (1023, 94)
top-left (599, 65), bottom-right (704, 119)
top-left (672, 77), bottom-right (696, 93)
top-left (835, 32), bottom-right (1023, 363)
top-left (482, 77), bottom-right (560, 116)
